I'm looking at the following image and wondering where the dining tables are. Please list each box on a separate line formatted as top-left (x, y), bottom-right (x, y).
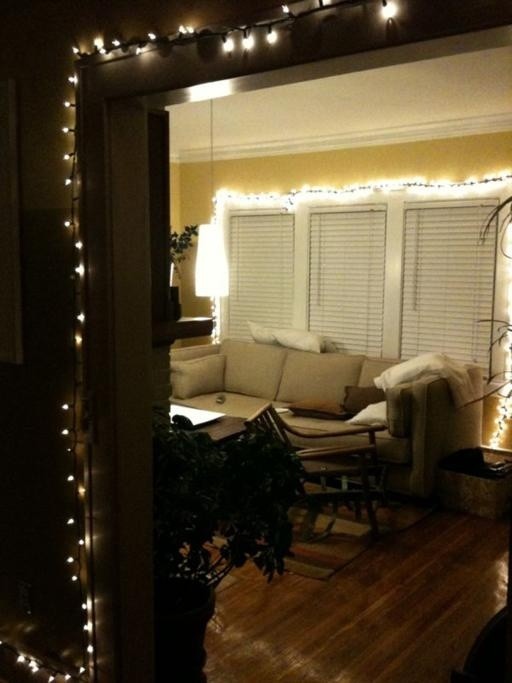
top-left (156, 415), bottom-right (299, 683)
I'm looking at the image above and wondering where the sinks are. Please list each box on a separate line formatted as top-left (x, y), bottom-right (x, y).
top-left (167, 338), bottom-right (485, 502)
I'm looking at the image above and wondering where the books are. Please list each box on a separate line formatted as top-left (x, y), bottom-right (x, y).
top-left (438, 446), bottom-right (512, 521)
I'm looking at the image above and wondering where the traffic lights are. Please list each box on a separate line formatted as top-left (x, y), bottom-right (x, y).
top-left (169, 353), bottom-right (227, 399)
top-left (248, 320), bottom-right (338, 355)
top-left (284, 383), bottom-right (388, 429)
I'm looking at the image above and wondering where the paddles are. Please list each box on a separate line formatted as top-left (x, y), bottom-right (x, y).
top-left (205, 478), bottom-right (436, 582)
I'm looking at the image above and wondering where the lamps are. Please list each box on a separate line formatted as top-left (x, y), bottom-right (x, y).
top-left (242, 403), bottom-right (392, 544)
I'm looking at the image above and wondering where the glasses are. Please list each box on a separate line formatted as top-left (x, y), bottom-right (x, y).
top-left (191, 97), bottom-right (230, 296)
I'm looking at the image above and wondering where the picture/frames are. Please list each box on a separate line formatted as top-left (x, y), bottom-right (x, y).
top-left (168, 402), bottom-right (249, 444)
